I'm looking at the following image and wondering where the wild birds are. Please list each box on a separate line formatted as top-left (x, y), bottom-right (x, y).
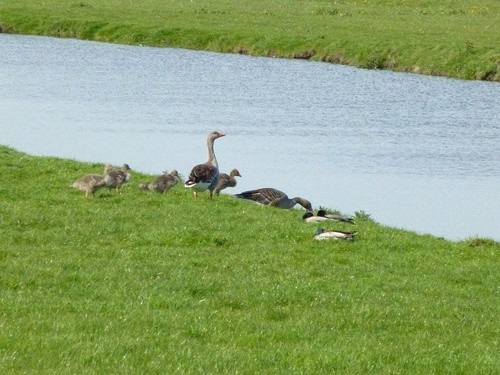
top-left (302, 212), bottom-right (338, 223)
top-left (104, 163), bottom-right (131, 193)
top-left (140, 169), bottom-right (180, 195)
top-left (70, 163), bottom-right (113, 197)
top-left (183, 131), bottom-right (226, 200)
top-left (317, 210), bottom-right (355, 224)
top-left (234, 187), bottom-right (314, 212)
top-left (209, 169), bottom-right (242, 200)
top-left (311, 227), bottom-right (358, 241)
